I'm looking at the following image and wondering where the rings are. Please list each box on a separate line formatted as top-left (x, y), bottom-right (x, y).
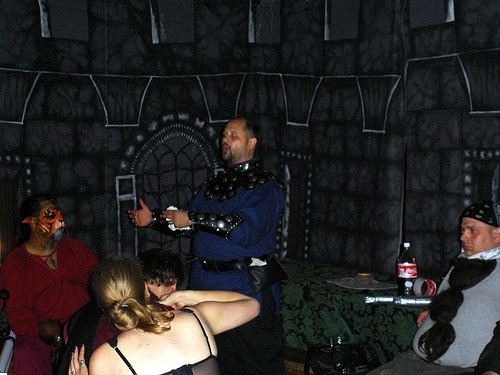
top-left (71, 370), bottom-right (75, 374)
top-left (80, 360), bottom-right (85, 367)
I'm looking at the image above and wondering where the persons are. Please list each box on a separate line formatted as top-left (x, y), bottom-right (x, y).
top-left (128, 115), bottom-right (285, 375)
top-left (68, 254), bottom-right (260, 375)
top-left (90, 248), bottom-right (179, 355)
top-left (365, 200), bottom-right (500, 375)
top-left (0, 195), bottom-right (102, 375)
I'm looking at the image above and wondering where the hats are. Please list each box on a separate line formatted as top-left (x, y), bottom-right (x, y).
top-left (460, 201), bottom-right (500, 227)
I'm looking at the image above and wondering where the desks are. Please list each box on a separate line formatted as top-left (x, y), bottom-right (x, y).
top-left (280, 258), bottom-right (440, 364)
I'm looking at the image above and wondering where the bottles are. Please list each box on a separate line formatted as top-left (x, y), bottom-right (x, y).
top-left (396, 242), bottom-right (418, 299)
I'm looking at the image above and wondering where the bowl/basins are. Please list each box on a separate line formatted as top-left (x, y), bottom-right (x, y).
top-left (355, 272), bottom-right (374, 286)
